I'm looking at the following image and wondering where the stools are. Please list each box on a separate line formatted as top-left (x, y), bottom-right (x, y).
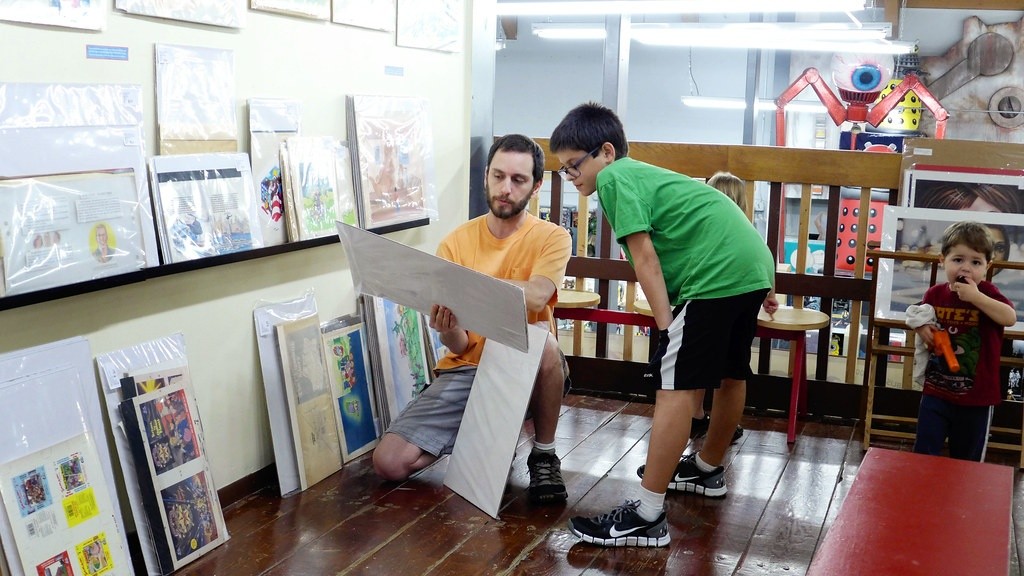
top-left (633, 296), bottom-right (678, 334)
top-left (758, 302), bottom-right (832, 446)
top-left (551, 288), bottom-right (603, 325)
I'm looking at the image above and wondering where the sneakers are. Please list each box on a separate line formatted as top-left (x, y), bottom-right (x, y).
top-left (690, 411), bottom-right (744, 443)
top-left (635, 450), bottom-right (727, 496)
top-left (525, 448), bottom-right (568, 504)
top-left (568, 499), bottom-right (671, 547)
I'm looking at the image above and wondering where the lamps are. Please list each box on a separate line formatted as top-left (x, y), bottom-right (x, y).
top-left (485, 0), bottom-right (933, 117)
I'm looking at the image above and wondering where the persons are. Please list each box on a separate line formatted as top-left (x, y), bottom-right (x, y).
top-left (922, 182), bottom-right (1017, 276)
top-left (372, 133), bottom-right (570, 507)
top-left (552, 104), bottom-right (777, 548)
top-left (914, 222), bottom-right (1015, 464)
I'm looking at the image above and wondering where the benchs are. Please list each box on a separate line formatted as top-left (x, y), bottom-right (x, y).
top-left (805, 445), bottom-right (1016, 576)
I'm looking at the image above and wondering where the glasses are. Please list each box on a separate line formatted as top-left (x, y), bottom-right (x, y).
top-left (557, 145), bottom-right (599, 181)
